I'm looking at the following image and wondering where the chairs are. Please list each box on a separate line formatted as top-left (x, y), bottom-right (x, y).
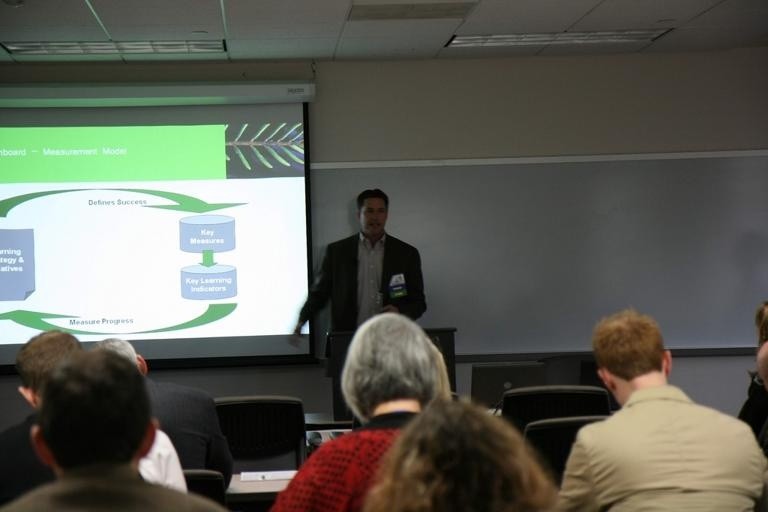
top-left (212, 394), bottom-right (308, 473)
top-left (499, 382), bottom-right (615, 491)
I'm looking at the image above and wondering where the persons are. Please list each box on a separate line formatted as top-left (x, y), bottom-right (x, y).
top-left (734, 301), bottom-right (768, 510)
top-left (557, 305), bottom-right (767, 510)
top-left (1, 331), bottom-right (184, 488)
top-left (290, 186), bottom-right (428, 421)
top-left (268, 308), bottom-right (459, 512)
top-left (361, 393), bottom-right (568, 511)
top-left (97, 337), bottom-right (235, 495)
top-left (0, 348), bottom-right (234, 512)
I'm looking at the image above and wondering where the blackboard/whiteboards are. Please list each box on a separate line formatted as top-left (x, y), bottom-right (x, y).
top-left (310, 151), bottom-right (766, 360)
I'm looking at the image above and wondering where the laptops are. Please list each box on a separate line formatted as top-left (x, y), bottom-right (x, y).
top-left (300, 421), bottom-right (355, 469)
